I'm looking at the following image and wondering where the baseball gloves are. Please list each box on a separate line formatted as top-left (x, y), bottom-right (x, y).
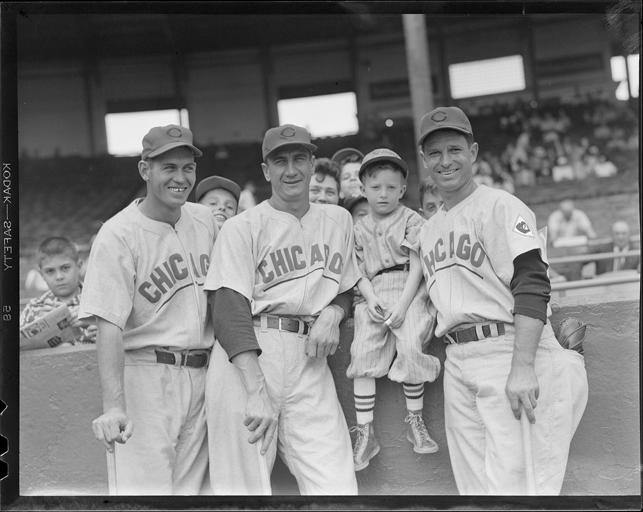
top-left (557, 318), bottom-right (587, 355)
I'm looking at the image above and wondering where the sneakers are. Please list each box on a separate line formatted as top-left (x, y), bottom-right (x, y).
top-left (403, 409), bottom-right (439, 454)
top-left (348, 420), bottom-right (381, 472)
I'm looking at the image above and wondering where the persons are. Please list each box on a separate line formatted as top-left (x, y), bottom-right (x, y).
top-left (203, 120), bottom-right (362, 497)
top-left (192, 86), bottom-right (635, 227)
top-left (346, 142), bottom-right (441, 472)
top-left (75, 122), bottom-right (219, 497)
top-left (592, 216), bottom-right (639, 273)
top-left (18, 234), bottom-right (96, 351)
top-left (414, 105), bottom-right (591, 497)
top-left (541, 198), bottom-right (602, 247)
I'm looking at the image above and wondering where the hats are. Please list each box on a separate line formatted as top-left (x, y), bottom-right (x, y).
top-left (262, 124), bottom-right (318, 163)
top-left (416, 106), bottom-right (474, 145)
top-left (195, 175), bottom-right (241, 203)
top-left (141, 124), bottom-right (203, 161)
top-left (331, 148), bottom-right (364, 163)
top-left (359, 148), bottom-right (408, 181)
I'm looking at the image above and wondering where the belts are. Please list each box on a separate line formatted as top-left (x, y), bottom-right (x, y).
top-left (154, 350), bottom-right (208, 368)
top-left (375, 264), bottom-right (410, 276)
top-left (442, 323), bottom-right (505, 345)
top-left (251, 315), bottom-right (308, 335)
